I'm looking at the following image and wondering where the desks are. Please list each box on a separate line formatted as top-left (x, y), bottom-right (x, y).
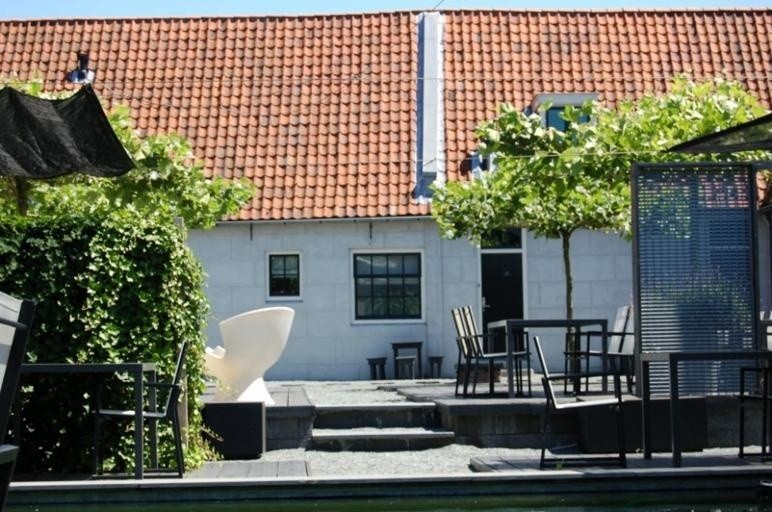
top-left (487, 318), bottom-right (609, 398)
top-left (639, 350), bottom-right (772, 467)
top-left (19, 363), bottom-right (158, 481)
top-left (388, 341), bottom-right (424, 379)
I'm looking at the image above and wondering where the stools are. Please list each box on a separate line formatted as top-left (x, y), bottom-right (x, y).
top-left (365, 356), bottom-right (444, 380)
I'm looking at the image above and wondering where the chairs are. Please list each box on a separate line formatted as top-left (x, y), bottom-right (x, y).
top-left (90, 341), bottom-right (188, 479)
top-left (532, 335), bottom-right (628, 470)
top-left (564, 305), bottom-right (635, 395)
top-left (450, 305), bottom-right (532, 399)
top-left (203, 306), bottom-right (295, 407)
top-left (738, 321), bottom-right (772, 462)
